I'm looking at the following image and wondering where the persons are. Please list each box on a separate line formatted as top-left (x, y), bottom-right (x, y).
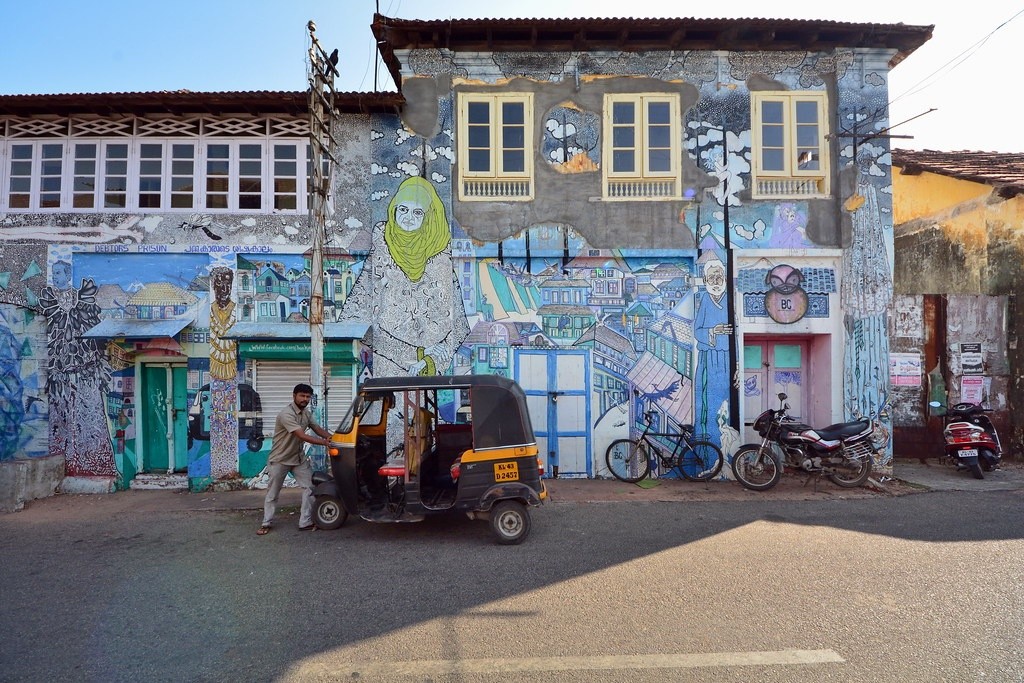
top-left (257, 384), bottom-right (333, 535)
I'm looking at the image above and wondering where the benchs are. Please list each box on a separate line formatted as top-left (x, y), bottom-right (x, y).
top-left (379, 406), bottom-right (430, 477)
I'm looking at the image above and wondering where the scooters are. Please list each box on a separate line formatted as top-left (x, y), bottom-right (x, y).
top-left (928, 394), bottom-right (1004, 480)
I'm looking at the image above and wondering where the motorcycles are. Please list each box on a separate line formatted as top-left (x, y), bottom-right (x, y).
top-left (731, 393), bottom-right (880, 494)
top-left (309, 374), bottom-right (548, 544)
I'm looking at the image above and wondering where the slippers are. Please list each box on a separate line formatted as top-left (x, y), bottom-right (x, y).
top-left (299, 523), bottom-right (319, 530)
top-left (257, 527), bottom-right (270, 535)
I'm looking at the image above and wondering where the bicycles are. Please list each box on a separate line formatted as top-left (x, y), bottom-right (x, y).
top-left (605, 409), bottom-right (724, 490)
